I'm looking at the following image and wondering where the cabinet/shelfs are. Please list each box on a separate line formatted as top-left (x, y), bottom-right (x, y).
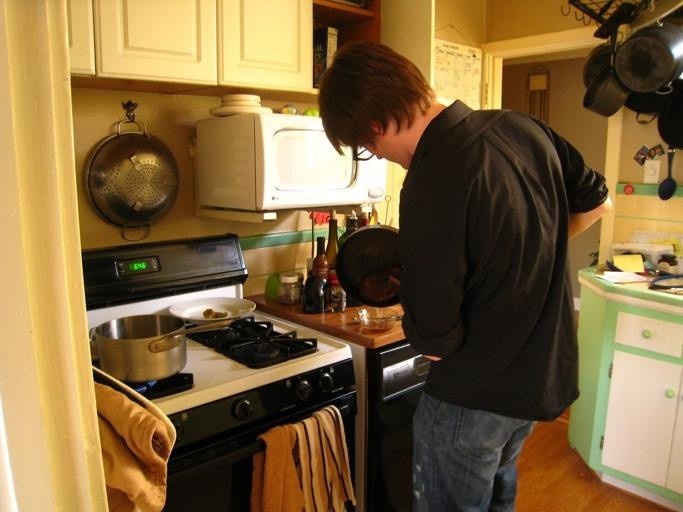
top-left (93, 0), bottom-right (312, 95)
top-left (313, 0), bottom-right (381, 98)
top-left (567, 263), bottom-right (683, 512)
top-left (68, 0), bottom-right (96, 90)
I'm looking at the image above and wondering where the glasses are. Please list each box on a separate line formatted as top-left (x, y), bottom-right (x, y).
top-left (352, 140), bottom-right (373, 162)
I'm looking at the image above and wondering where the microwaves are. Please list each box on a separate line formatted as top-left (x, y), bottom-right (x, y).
top-left (196, 112), bottom-right (387, 212)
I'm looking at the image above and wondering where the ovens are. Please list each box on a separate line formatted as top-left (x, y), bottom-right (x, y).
top-left (161, 390), bottom-right (358, 512)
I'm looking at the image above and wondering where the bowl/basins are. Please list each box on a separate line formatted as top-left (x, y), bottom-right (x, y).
top-left (358, 307), bottom-right (397, 333)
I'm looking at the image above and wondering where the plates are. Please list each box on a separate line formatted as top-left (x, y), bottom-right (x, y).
top-left (168, 297), bottom-right (257, 325)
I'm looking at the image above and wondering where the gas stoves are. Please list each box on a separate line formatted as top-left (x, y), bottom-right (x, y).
top-left (94, 309), bottom-right (356, 456)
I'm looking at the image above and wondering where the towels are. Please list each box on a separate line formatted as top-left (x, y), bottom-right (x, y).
top-left (294, 404), bottom-right (357, 512)
top-left (250, 423), bottom-right (306, 512)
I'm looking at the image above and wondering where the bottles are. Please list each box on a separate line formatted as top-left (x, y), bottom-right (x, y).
top-left (277, 207), bottom-right (370, 314)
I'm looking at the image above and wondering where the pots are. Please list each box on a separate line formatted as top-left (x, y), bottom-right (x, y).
top-left (89, 314), bottom-right (234, 384)
top-left (582, 20), bottom-right (682, 149)
top-left (335, 224), bottom-right (401, 309)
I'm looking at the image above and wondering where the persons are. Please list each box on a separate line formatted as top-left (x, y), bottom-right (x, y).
top-left (319, 40), bottom-right (613, 512)
top-left (649, 146), bottom-right (664, 160)
